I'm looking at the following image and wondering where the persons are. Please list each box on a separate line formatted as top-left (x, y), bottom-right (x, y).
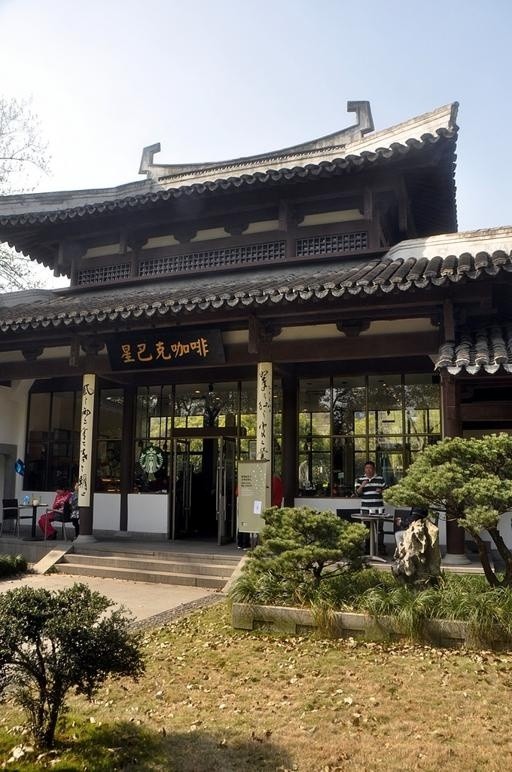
top-left (65, 480), bottom-right (80, 541)
top-left (354, 461), bottom-right (390, 557)
top-left (391, 500), bottom-right (431, 562)
top-left (39, 485), bottom-right (72, 540)
top-left (268, 469), bottom-right (284, 510)
top-left (171, 461), bottom-right (236, 538)
top-left (235, 482), bottom-right (253, 551)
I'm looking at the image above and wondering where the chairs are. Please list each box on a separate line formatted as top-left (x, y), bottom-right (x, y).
top-left (39, 502), bottom-right (74, 544)
top-left (335, 508), bottom-right (411, 556)
top-left (2, 497), bottom-right (34, 539)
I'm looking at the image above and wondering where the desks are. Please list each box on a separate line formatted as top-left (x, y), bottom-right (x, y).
top-left (349, 512), bottom-right (392, 562)
top-left (16, 504), bottom-right (49, 541)
